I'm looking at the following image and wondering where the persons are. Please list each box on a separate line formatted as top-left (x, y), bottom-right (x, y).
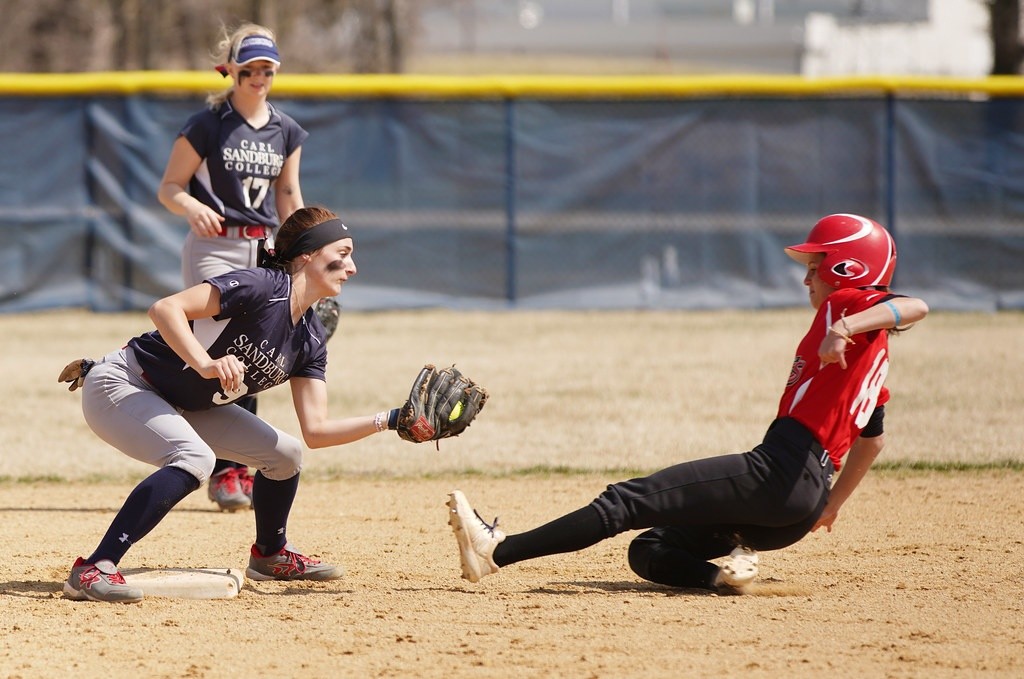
top-left (157, 23), bottom-right (309, 512)
top-left (446, 213), bottom-right (929, 596)
top-left (63, 207), bottom-right (489, 603)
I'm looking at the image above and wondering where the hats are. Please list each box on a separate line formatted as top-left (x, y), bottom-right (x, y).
top-left (228, 35), bottom-right (281, 67)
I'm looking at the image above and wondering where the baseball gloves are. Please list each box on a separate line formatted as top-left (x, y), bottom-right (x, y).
top-left (396, 362), bottom-right (491, 444)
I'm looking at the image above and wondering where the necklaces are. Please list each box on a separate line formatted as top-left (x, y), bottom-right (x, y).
top-left (291, 284), bottom-right (320, 344)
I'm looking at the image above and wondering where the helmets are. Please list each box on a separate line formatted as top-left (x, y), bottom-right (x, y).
top-left (785, 213), bottom-right (897, 289)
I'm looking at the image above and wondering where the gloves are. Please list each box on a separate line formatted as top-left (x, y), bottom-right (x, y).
top-left (58, 358), bottom-right (95, 391)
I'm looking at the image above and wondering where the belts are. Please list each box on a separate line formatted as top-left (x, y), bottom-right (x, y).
top-left (811, 441), bottom-right (830, 468)
top-left (122, 345), bottom-right (159, 388)
top-left (217, 224), bottom-right (273, 240)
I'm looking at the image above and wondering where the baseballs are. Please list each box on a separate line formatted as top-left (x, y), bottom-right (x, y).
top-left (448, 400), bottom-right (465, 420)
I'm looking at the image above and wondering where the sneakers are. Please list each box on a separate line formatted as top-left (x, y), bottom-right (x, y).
top-left (209, 467), bottom-right (255, 513)
top-left (716, 545), bottom-right (758, 587)
top-left (246, 542), bottom-right (343, 581)
top-left (63, 557), bottom-right (144, 603)
top-left (445, 490), bottom-right (505, 583)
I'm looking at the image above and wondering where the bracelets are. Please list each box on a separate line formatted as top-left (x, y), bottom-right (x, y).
top-left (374, 412), bottom-right (386, 433)
top-left (885, 301), bottom-right (901, 326)
top-left (827, 313), bottom-right (855, 344)
